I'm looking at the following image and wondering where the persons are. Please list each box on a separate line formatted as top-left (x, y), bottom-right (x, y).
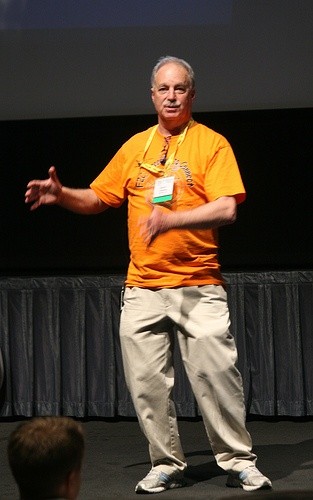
top-left (24, 57), bottom-right (273, 493)
top-left (7, 416), bottom-right (87, 500)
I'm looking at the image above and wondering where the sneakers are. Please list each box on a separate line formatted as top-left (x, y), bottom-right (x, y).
top-left (227, 468), bottom-right (272, 492)
top-left (135, 469), bottom-right (187, 493)
